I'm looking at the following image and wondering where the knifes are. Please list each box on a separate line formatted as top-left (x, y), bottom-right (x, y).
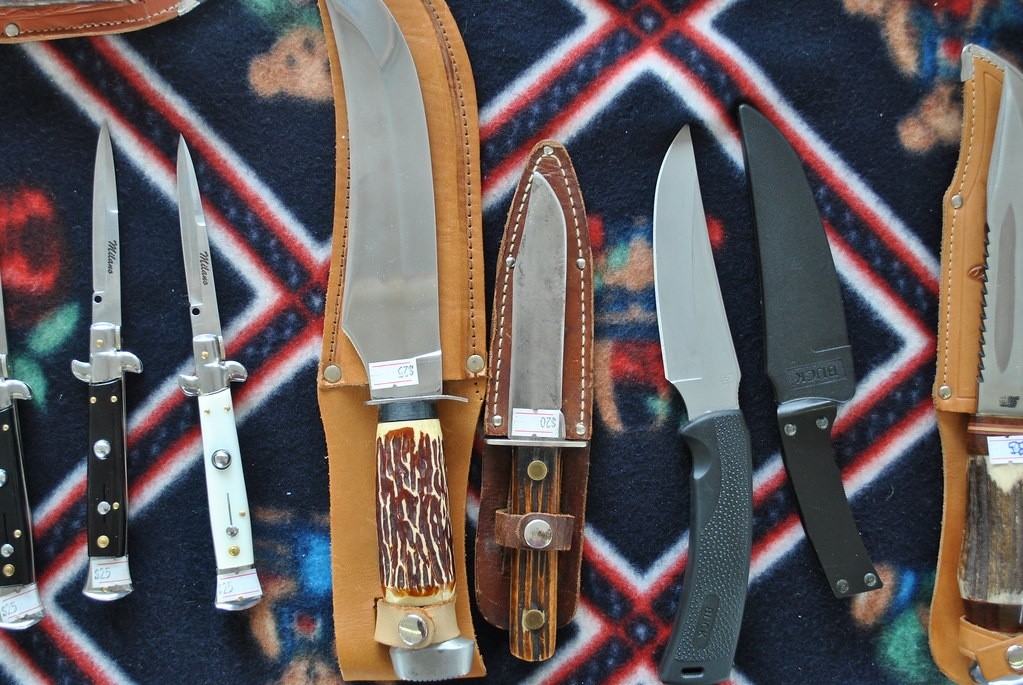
top-left (0, 278), bottom-right (45, 628)
top-left (70, 121), bottom-right (144, 603)
top-left (737, 103), bottom-right (883, 597)
top-left (959, 67), bottom-right (1023, 606)
top-left (323, 0), bottom-right (472, 683)
top-left (175, 133), bottom-right (264, 609)
top-left (653, 122), bottom-right (752, 685)
top-left (485, 171), bottom-right (588, 663)
